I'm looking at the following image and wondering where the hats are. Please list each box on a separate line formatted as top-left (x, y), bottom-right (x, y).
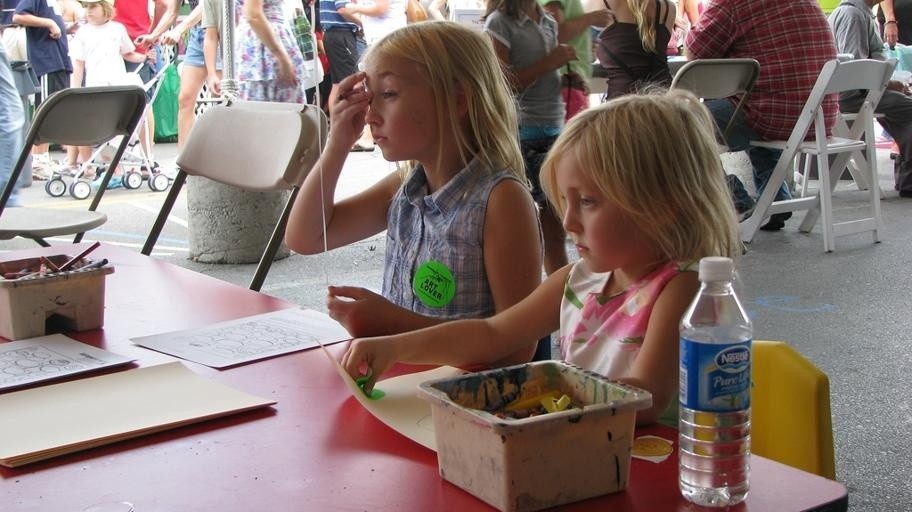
top-left (74, 0), bottom-right (114, 4)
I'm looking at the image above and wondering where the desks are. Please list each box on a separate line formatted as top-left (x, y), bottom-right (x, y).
top-left (589, 57), bottom-right (687, 98)
top-left (0, 242), bottom-right (849, 512)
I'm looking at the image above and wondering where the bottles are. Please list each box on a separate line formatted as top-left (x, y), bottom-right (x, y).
top-left (677, 256), bottom-right (752, 506)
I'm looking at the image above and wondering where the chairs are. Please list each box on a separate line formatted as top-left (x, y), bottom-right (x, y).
top-left (0, 85), bottom-right (147, 246)
top-left (752, 341), bottom-right (836, 481)
top-left (667, 59), bottom-right (762, 255)
top-left (534, 336), bottom-right (548, 362)
top-left (841, 112), bottom-right (890, 198)
top-left (743, 59), bottom-right (898, 253)
top-left (142, 99), bottom-right (328, 291)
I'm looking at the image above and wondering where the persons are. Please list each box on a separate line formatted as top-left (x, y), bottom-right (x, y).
top-left (479, 0), bottom-right (911, 279)
top-left (339, 86), bottom-right (740, 431)
top-left (0, 1), bottom-right (450, 206)
top-left (284, 20), bottom-right (551, 373)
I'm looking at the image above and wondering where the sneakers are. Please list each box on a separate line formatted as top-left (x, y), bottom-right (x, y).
top-left (31, 162), bottom-right (52, 181)
top-left (760, 211), bottom-right (793, 230)
top-left (349, 139), bottom-right (376, 152)
top-left (47, 160), bottom-right (60, 175)
top-left (728, 200), bottom-right (756, 222)
top-left (60, 165), bottom-right (82, 179)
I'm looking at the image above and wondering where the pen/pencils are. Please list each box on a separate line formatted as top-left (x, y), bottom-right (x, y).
top-left (40, 255), bottom-right (61, 272)
top-left (5, 272), bottom-right (30, 280)
top-left (75, 258), bottom-right (108, 270)
top-left (59, 241), bottom-right (100, 272)
top-left (17, 271), bottom-right (39, 279)
top-left (40, 264), bottom-right (46, 273)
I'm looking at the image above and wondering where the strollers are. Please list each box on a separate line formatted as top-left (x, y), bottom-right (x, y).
top-left (45, 36), bottom-right (180, 200)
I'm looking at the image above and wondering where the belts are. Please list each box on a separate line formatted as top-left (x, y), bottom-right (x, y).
top-left (330, 27), bottom-right (354, 34)
